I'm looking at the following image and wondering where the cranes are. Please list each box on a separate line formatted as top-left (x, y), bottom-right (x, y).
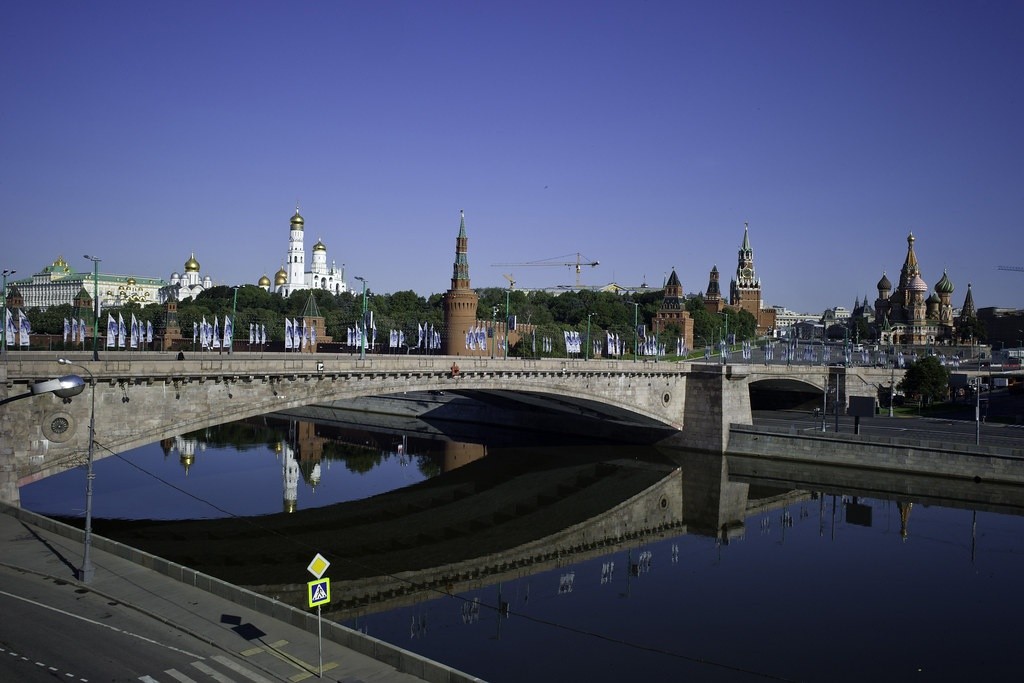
top-left (490, 251), bottom-right (599, 292)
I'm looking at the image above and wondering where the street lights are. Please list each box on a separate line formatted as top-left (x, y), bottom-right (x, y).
top-left (84, 255), bottom-right (102, 361)
top-left (977, 364), bottom-right (985, 443)
top-left (354, 276), bottom-right (368, 359)
top-left (698, 336), bottom-right (707, 365)
top-left (59, 358), bottom-right (96, 583)
top-left (0, 269), bottom-right (17, 353)
